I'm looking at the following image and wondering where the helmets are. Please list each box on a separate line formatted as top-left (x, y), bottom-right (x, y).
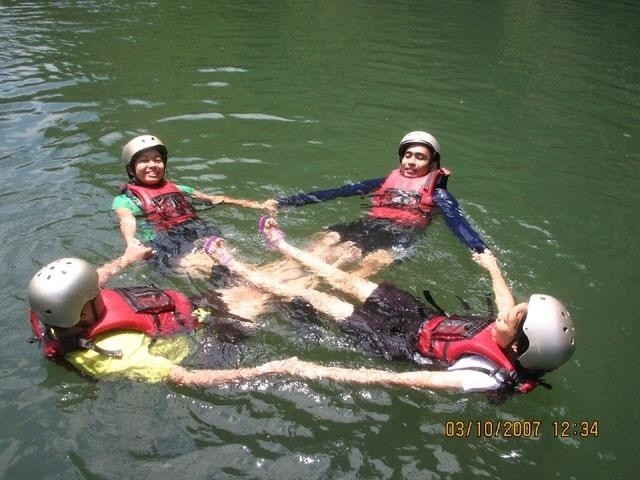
top-left (122, 135), bottom-right (167, 167)
top-left (29, 257), bottom-right (99, 329)
top-left (517, 294), bottom-right (575, 375)
top-left (399, 131), bottom-right (440, 159)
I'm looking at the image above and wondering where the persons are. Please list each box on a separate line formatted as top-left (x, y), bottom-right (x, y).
top-left (24, 230), bottom-right (364, 392)
top-left (202, 215), bottom-right (577, 392)
top-left (260, 129), bottom-right (492, 280)
top-left (109, 133), bottom-right (276, 285)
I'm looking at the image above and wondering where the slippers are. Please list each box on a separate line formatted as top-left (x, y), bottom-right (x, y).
top-left (259, 215), bottom-right (285, 244)
top-left (204, 236), bottom-right (231, 265)
